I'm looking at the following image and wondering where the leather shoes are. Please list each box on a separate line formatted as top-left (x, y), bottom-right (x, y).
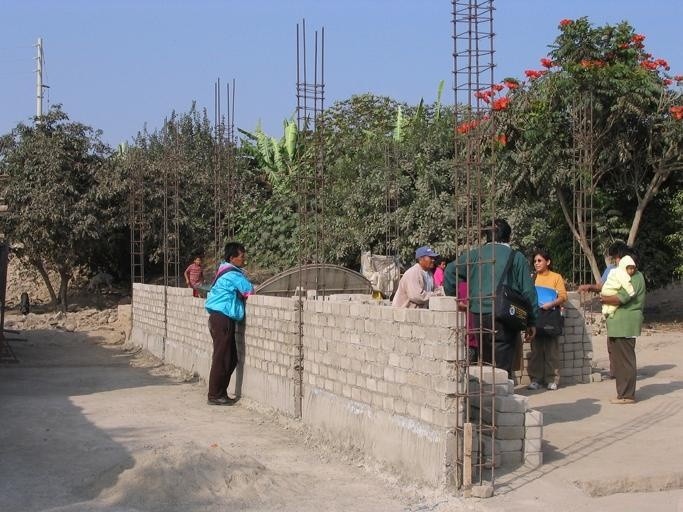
top-left (205, 397), bottom-right (235, 406)
top-left (609, 398), bottom-right (634, 405)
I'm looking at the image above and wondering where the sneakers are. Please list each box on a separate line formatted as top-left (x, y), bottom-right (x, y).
top-left (528, 382), bottom-right (544, 390)
top-left (546, 382), bottom-right (557, 390)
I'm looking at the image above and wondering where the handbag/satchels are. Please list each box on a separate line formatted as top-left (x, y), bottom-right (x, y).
top-left (532, 307), bottom-right (562, 338)
top-left (494, 283), bottom-right (527, 331)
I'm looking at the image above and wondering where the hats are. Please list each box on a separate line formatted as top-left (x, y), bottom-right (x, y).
top-left (414, 245), bottom-right (439, 259)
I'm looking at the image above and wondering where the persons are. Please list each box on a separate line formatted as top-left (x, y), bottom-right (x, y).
top-left (578, 242), bottom-right (646, 404)
top-left (395, 246), bottom-right (478, 362)
top-left (526, 250), bottom-right (568, 390)
top-left (443, 217), bottom-right (539, 379)
top-left (205, 240), bottom-right (256, 407)
top-left (183, 257), bottom-right (204, 287)
